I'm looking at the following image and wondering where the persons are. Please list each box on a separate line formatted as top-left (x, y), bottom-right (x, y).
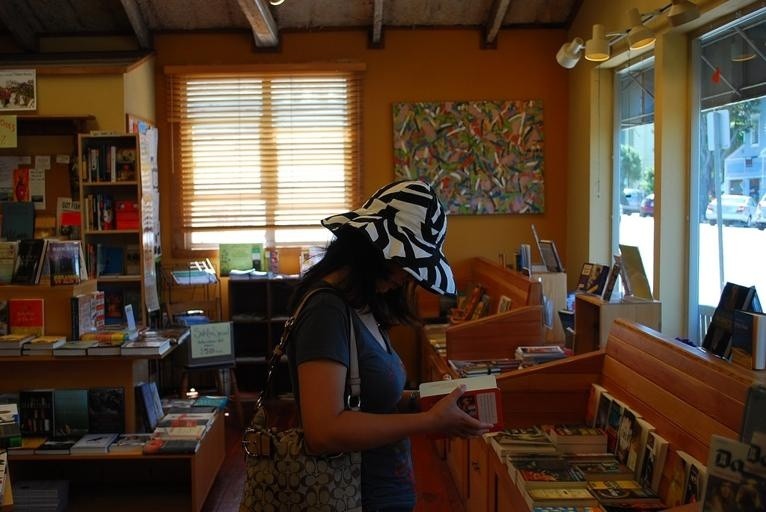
top-left (242, 179), bottom-right (497, 507)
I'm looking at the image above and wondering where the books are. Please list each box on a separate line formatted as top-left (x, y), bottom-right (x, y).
top-left (539, 240), bottom-right (563, 272)
top-left (416, 373), bottom-right (503, 434)
top-left (510, 251), bottom-right (522, 270)
top-left (488, 387), bottom-right (765, 512)
top-left (85, 138), bottom-right (139, 233)
top-left (420, 286), bottom-right (514, 359)
top-left (731, 309), bottom-right (765, 374)
top-left (445, 344), bottom-right (572, 379)
top-left (0, 237), bottom-right (267, 458)
top-left (522, 245), bottom-right (534, 272)
top-left (575, 263), bottom-right (620, 303)
top-left (700, 281), bottom-right (755, 360)
top-left (12, 481), bottom-right (68, 512)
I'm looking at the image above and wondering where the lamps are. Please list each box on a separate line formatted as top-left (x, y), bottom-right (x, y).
top-left (554, 1), bottom-right (703, 71)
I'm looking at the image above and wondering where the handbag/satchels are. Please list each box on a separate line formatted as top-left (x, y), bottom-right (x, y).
top-left (238, 407), bottom-right (363, 512)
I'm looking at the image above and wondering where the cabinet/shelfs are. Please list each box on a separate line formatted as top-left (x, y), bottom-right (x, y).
top-left (227, 275), bottom-right (307, 427)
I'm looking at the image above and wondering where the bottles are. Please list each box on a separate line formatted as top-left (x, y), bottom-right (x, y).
top-left (253, 245), bottom-right (262, 271)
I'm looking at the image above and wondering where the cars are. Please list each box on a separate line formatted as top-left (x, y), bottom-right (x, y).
top-left (640, 191), bottom-right (654, 218)
top-left (620, 189), bottom-right (648, 216)
top-left (706, 195), bottom-right (757, 227)
top-left (753, 195), bottom-right (766, 230)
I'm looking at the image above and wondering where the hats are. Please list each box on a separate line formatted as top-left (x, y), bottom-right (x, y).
top-left (321, 180), bottom-right (458, 298)
